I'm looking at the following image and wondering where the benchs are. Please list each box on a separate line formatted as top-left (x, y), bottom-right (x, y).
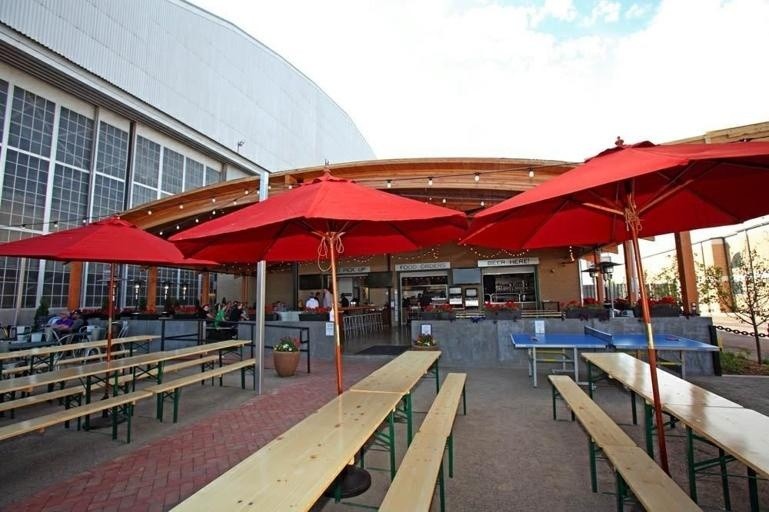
top-left (419, 373), bottom-right (467, 476)
top-left (547, 374), bottom-right (637, 492)
top-left (602, 445), bottom-right (703, 512)
top-left (1, 334), bottom-right (258, 442)
top-left (378, 433), bottom-right (447, 512)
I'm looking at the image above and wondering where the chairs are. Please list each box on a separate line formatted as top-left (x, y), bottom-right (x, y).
top-left (1, 317), bottom-right (131, 398)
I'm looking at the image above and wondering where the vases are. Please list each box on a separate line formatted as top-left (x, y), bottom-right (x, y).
top-left (299, 314), bottom-right (329, 321)
top-left (633, 307), bottom-right (679, 317)
top-left (273, 352), bottom-right (300, 377)
top-left (485, 311), bottom-right (524, 319)
top-left (566, 312), bottom-right (606, 318)
top-left (249, 314), bottom-right (278, 320)
top-left (423, 312), bottom-right (454, 319)
top-left (413, 344), bottom-right (439, 370)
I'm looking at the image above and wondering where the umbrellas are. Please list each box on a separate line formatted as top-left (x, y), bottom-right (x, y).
top-left (459, 135), bottom-right (769, 476)
top-left (167, 166), bottom-right (468, 395)
top-left (0, 215), bottom-right (219, 417)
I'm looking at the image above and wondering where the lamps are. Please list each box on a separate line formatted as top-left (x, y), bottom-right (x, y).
top-left (580, 261), bottom-right (622, 278)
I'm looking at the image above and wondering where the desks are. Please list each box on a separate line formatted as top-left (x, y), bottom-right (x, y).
top-left (339, 306), bottom-right (379, 313)
top-left (581, 352), bottom-right (744, 459)
top-left (511, 325), bottom-right (722, 389)
top-left (663, 404), bottom-right (769, 512)
top-left (349, 351), bottom-right (442, 445)
top-left (167, 390), bottom-right (411, 512)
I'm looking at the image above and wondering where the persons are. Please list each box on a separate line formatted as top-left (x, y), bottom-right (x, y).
top-left (200, 297), bottom-right (256, 330)
top-left (299, 285), bottom-right (359, 308)
top-left (52, 310), bottom-right (74, 346)
top-left (402, 287), bottom-right (445, 310)
top-left (507, 283), bottom-right (518, 293)
top-left (273, 301), bottom-right (281, 321)
top-left (60, 309), bottom-right (83, 354)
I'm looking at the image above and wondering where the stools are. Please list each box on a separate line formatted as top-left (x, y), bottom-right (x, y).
top-left (342, 312), bottom-right (384, 339)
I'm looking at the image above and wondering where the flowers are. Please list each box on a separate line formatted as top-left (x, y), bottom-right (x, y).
top-left (249, 303), bottom-right (273, 314)
top-left (481, 301), bottom-right (519, 313)
top-left (561, 300), bottom-right (603, 313)
top-left (637, 297), bottom-right (676, 307)
top-left (423, 304), bottom-right (452, 312)
top-left (413, 333), bottom-right (437, 346)
top-left (273, 336), bottom-right (301, 352)
top-left (302, 307), bottom-right (328, 314)
